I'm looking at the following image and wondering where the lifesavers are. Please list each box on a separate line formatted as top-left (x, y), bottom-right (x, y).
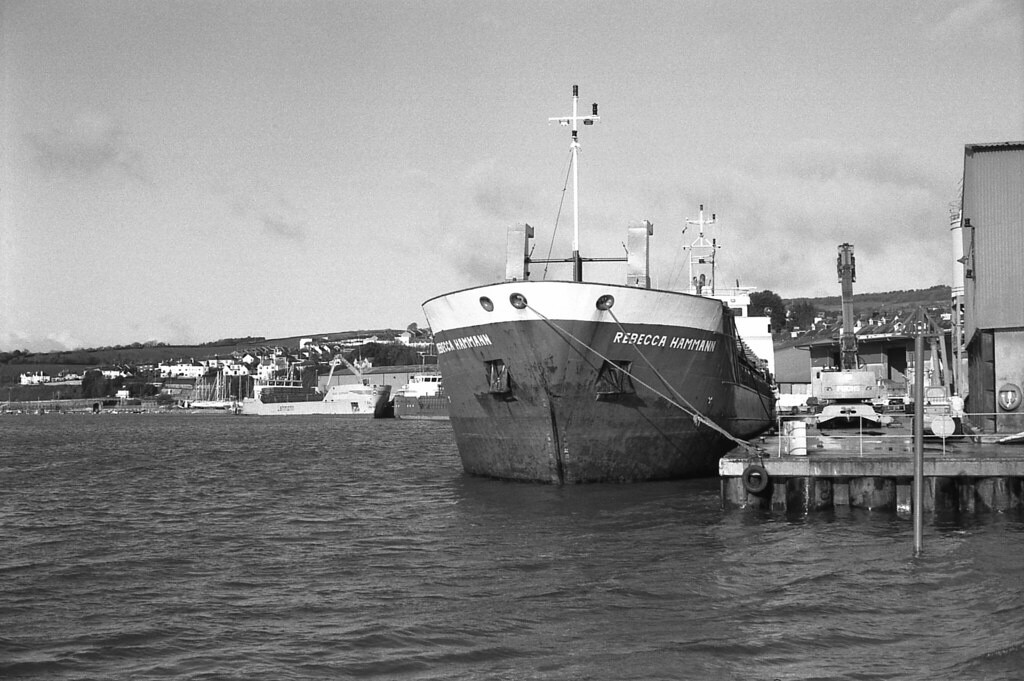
top-left (741, 464), bottom-right (770, 494)
top-left (792, 406), bottom-right (799, 415)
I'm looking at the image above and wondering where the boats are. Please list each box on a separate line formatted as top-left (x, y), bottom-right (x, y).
top-left (421, 86), bottom-right (775, 488)
top-left (235, 352), bottom-right (391, 420)
top-left (393, 376), bottom-right (450, 421)
